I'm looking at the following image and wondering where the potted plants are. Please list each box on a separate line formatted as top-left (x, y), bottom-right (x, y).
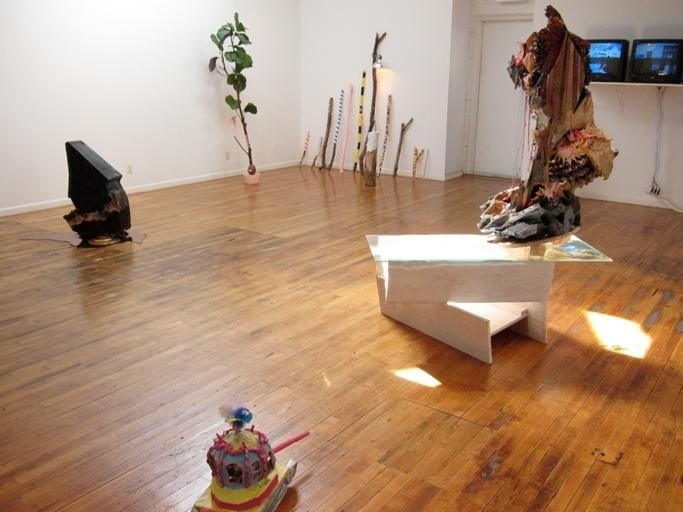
top-left (207, 11), bottom-right (268, 183)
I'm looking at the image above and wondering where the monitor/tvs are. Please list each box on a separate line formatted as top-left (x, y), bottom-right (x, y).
top-left (627, 38), bottom-right (683, 84)
top-left (584, 38), bottom-right (630, 82)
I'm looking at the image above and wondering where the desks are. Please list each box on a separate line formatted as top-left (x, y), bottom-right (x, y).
top-left (365, 231), bottom-right (617, 363)
